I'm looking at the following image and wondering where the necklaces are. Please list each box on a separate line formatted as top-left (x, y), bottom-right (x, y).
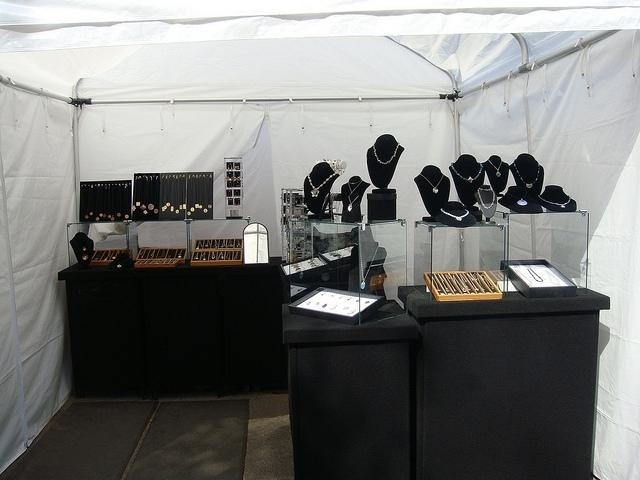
top-left (345, 182), bottom-right (364, 195)
top-left (513, 162), bottom-right (540, 189)
top-left (307, 172), bottom-right (337, 198)
top-left (508, 194), bottom-right (532, 206)
top-left (74, 171), bottom-right (244, 269)
top-left (420, 172), bottom-right (445, 194)
top-left (372, 143), bottom-right (399, 166)
top-left (355, 242), bottom-right (381, 289)
top-left (488, 159), bottom-right (504, 179)
top-left (524, 264), bottom-right (544, 285)
top-left (538, 194), bottom-right (572, 209)
top-left (441, 207), bottom-right (470, 222)
top-left (450, 162), bottom-right (484, 184)
top-left (347, 194), bottom-right (361, 212)
top-left (478, 188), bottom-right (496, 210)
